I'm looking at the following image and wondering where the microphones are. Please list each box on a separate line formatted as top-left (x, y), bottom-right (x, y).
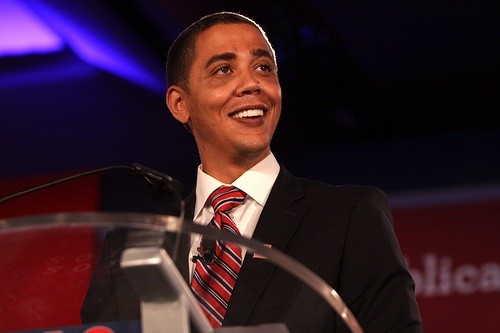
top-left (0, 164), bottom-right (172, 204)
top-left (203, 235), bottom-right (216, 262)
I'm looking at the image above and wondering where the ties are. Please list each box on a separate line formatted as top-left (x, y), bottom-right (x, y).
top-left (190, 185), bottom-right (249, 328)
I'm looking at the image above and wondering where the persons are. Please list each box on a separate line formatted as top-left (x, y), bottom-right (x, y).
top-left (80, 11), bottom-right (424, 332)
